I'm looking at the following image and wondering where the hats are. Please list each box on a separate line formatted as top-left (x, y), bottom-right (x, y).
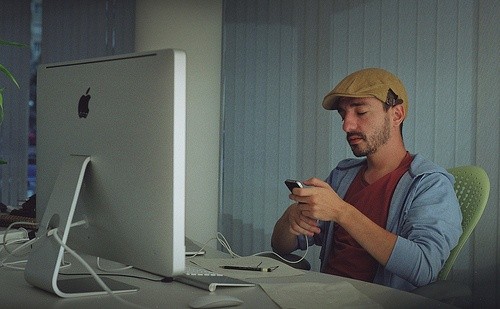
top-left (322, 68), bottom-right (408, 121)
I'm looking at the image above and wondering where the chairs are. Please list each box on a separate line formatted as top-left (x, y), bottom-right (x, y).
top-left (410, 166), bottom-right (491, 309)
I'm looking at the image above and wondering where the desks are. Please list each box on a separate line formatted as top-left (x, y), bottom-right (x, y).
top-left (0, 239), bottom-right (462, 309)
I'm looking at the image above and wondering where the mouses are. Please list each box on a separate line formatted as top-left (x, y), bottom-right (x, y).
top-left (188, 294), bottom-right (243, 309)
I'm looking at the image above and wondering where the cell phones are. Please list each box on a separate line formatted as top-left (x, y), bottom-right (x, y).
top-left (285, 179), bottom-right (304, 194)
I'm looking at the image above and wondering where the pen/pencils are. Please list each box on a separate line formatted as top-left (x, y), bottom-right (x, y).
top-left (220, 266), bottom-right (271, 272)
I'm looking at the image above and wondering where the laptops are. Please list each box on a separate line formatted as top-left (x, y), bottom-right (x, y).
top-left (185, 236), bottom-right (206, 256)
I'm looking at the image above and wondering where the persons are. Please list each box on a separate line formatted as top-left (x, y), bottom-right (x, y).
top-left (271, 68), bottom-right (463, 289)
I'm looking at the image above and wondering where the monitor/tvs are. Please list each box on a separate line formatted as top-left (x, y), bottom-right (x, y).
top-left (23, 48), bottom-right (187, 299)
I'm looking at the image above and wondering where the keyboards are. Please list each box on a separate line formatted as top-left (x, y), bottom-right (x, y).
top-left (173, 262), bottom-right (256, 292)
